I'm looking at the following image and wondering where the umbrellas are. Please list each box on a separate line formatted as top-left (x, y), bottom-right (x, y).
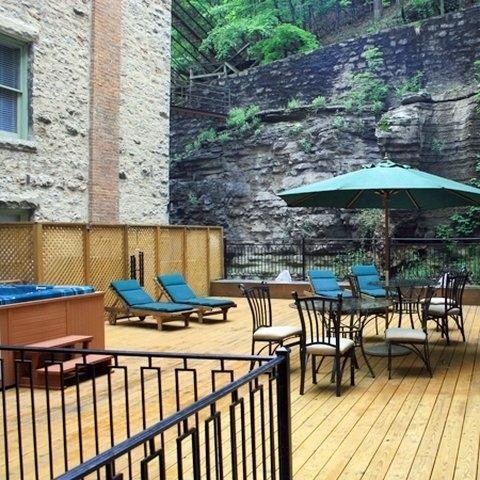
top-left (274, 156), bottom-right (480, 330)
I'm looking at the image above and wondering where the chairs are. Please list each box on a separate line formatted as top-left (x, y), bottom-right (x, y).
top-left (346, 273), bottom-right (390, 337)
top-left (290, 289), bottom-right (358, 397)
top-left (420, 269), bottom-right (466, 332)
top-left (423, 272), bottom-right (471, 347)
top-left (104, 279), bottom-right (199, 332)
top-left (233, 281), bottom-right (303, 372)
top-left (303, 270), bottom-right (352, 301)
top-left (151, 272), bottom-right (237, 324)
top-left (385, 286), bottom-right (438, 381)
top-left (346, 265), bottom-right (400, 303)
